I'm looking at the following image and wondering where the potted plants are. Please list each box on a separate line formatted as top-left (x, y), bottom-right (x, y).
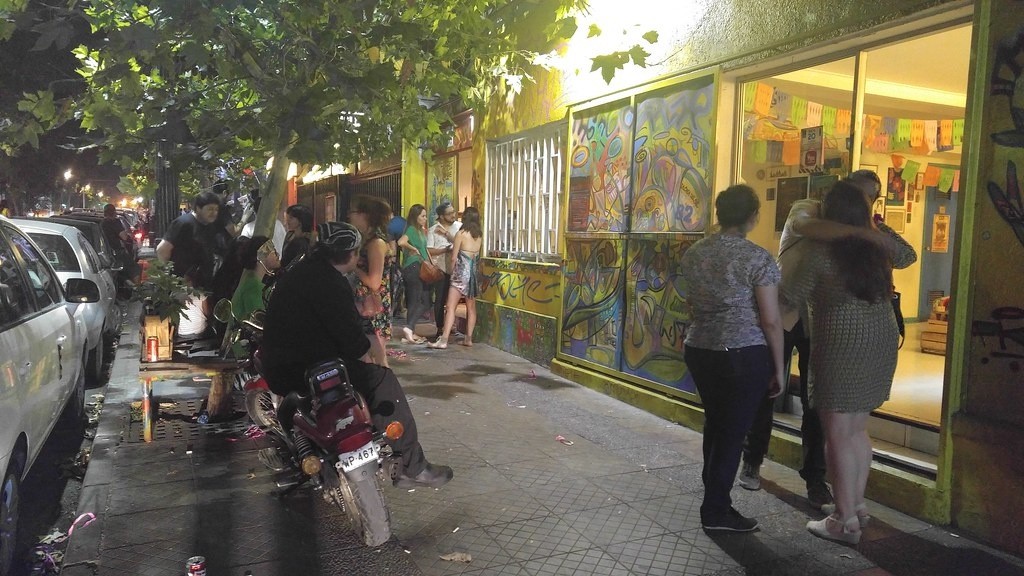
top-left (130, 255), bottom-right (212, 363)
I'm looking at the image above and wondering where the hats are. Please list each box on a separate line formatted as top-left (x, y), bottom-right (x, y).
top-left (435, 201), bottom-right (452, 220)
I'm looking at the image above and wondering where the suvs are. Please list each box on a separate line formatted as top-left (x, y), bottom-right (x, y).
top-left (0, 212), bottom-right (102, 576)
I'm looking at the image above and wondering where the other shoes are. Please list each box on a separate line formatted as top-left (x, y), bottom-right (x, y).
top-left (428, 336), bottom-right (447, 349)
top-left (450, 324), bottom-right (459, 336)
top-left (413, 333), bottom-right (427, 342)
top-left (458, 335), bottom-right (472, 346)
top-left (401, 337), bottom-right (423, 345)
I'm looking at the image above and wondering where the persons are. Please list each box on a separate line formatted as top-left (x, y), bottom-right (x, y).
top-left (156, 178), bottom-right (262, 340)
top-left (350, 194), bottom-right (393, 368)
top-left (682, 182), bottom-right (784, 531)
top-left (222, 235), bottom-right (280, 357)
top-left (739, 169), bottom-right (918, 544)
top-left (102, 204), bottom-right (133, 271)
top-left (261, 222), bottom-right (453, 488)
top-left (398, 204), bottom-right (483, 349)
top-left (282, 204), bottom-right (323, 256)
top-left (182, 208), bottom-right (187, 215)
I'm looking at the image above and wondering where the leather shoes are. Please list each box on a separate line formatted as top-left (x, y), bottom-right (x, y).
top-left (393, 459), bottom-right (453, 488)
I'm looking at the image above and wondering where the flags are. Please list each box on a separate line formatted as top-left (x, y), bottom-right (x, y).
top-left (243, 167), bottom-right (253, 175)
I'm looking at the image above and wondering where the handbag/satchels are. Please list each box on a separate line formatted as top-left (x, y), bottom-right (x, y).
top-left (418, 249), bottom-right (444, 284)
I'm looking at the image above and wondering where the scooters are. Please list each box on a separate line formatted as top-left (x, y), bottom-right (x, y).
top-left (213, 297), bottom-right (404, 552)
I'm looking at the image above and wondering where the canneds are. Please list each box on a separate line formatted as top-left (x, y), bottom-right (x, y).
top-left (185, 555), bottom-right (207, 576)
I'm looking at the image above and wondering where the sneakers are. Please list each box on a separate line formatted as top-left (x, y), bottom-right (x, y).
top-left (703, 508), bottom-right (758, 531)
top-left (738, 462), bottom-right (760, 490)
top-left (808, 484), bottom-right (833, 507)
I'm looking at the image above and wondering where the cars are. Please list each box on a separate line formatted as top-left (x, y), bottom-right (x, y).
top-left (9, 208), bottom-right (145, 383)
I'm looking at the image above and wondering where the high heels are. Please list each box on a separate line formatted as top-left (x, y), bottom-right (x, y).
top-left (821, 503), bottom-right (869, 527)
top-left (806, 513), bottom-right (861, 545)
